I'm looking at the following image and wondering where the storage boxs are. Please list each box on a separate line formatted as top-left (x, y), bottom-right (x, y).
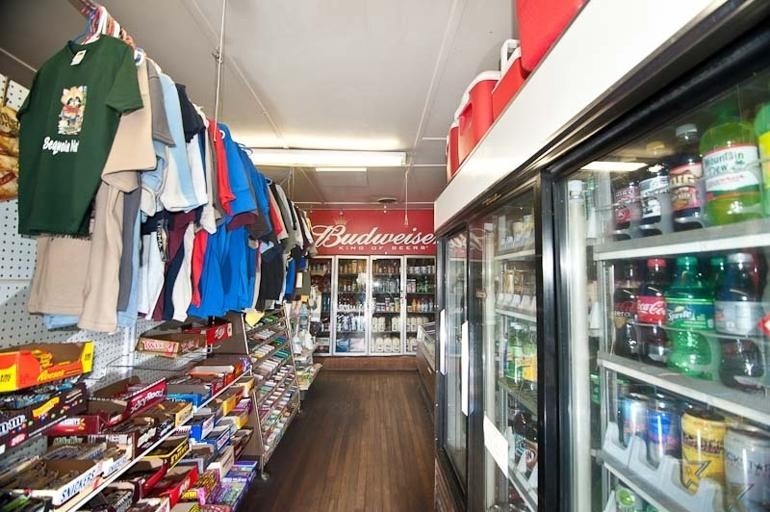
top-left (446, 121), bottom-right (459, 181)
top-left (453, 70), bottom-right (501, 163)
top-left (490, 39), bottom-right (527, 121)
top-left (514, 1), bottom-right (584, 71)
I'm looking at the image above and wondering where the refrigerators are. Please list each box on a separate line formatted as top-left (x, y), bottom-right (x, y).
top-left (433, 0), bottom-right (770, 512)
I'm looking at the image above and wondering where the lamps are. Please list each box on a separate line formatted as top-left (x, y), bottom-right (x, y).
top-left (236, 146), bottom-right (410, 170)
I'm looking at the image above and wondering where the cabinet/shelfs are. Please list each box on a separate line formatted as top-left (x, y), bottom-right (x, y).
top-left (208, 302), bottom-right (301, 474)
top-left (1, 357), bottom-right (258, 512)
top-left (284, 301), bottom-right (313, 401)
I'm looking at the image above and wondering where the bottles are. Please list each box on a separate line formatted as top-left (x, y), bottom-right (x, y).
top-left (507, 396), bottom-right (539, 480)
top-left (322, 296), bottom-right (330, 311)
top-left (614, 248), bottom-right (770, 393)
top-left (321, 317), bottom-right (329, 331)
top-left (505, 321), bottom-right (537, 395)
top-left (611, 98), bottom-right (770, 241)
top-left (337, 259), bottom-right (434, 353)
top-left (589, 342), bottom-right (630, 406)
top-left (309, 261), bottom-right (330, 273)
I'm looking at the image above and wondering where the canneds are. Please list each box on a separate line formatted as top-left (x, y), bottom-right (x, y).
top-left (620, 392), bottom-right (769, 511)
